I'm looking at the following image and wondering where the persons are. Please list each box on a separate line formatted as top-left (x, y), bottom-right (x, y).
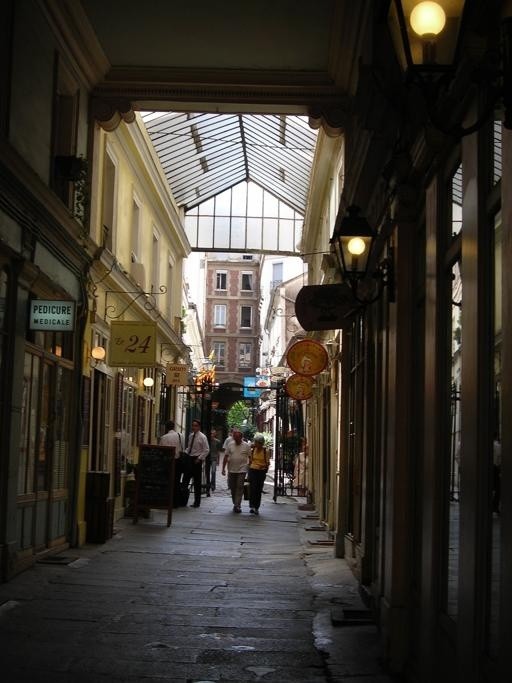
top-left (222, 428), bottom-right (252, 513)
top-left (208, 428), bottom-right (222, 491)
top-left (181, 418), bottom-right (210, 508)
top-left (222, 426), bottom-right (238, 489)
top-left (247, 432), bottom-right (270, 515)
top-left (159, 419), bottom-right (186, 482)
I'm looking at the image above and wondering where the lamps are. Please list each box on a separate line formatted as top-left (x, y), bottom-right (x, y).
top-left (142, 377), bottom-right (155, 400)
top-left (86, 347), bottom-right (105, 369)
top-left (387, 0), bottom-right (512, 133)
top-left (329, 205), bottom-right (395, 308)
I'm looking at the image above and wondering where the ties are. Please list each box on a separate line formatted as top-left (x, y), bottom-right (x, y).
top-left (188, 433), bottom-right (196, 453)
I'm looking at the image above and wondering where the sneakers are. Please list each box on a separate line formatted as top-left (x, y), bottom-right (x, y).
top-left (187, 502), bottom-right (263, 515)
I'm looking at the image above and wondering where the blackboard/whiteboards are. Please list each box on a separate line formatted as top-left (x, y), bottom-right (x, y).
top-left (134, 444), bottom-right (176, 510)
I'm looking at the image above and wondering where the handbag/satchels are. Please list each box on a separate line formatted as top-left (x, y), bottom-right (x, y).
top-left (243, 483), bottom-right (251, 499)
